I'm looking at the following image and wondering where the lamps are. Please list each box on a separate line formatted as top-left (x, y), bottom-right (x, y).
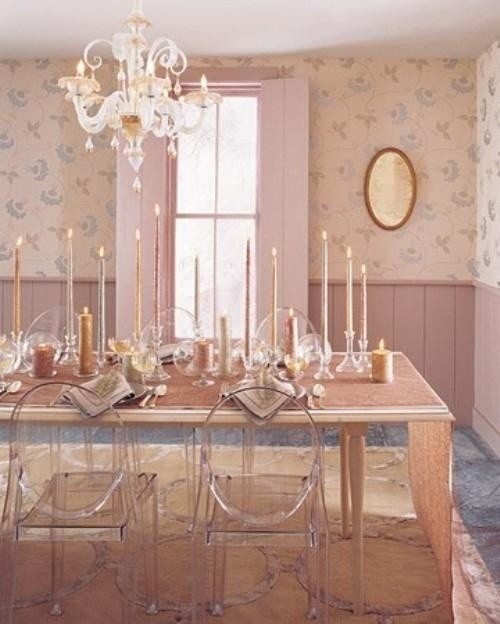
top-left (61, 1), bottom-right (224, 197)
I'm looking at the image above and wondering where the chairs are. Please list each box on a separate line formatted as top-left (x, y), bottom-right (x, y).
top-left (183, 384), bottom-right (333, 619)
top-left (4, 380), bottom-right (164, 618)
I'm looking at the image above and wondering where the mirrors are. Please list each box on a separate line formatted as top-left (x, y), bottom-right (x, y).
top-left (365, 147), bottom-right (418, 230)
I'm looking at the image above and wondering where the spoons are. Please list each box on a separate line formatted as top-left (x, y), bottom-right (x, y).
top-left (313, 384), bottom-right (326, 410)
top-left (0, 381), bottom-right (22, 402)
top-left (147, 383), bottom-right (168, 408)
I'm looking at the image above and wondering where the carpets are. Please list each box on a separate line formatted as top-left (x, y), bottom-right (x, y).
top-left (2, 441), bottom-right (500, 624)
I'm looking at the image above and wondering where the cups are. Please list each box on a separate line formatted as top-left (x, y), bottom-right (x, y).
top-left (106, 333), bottom-right (305, 382)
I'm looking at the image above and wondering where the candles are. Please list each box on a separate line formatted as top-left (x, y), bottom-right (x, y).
top-left (12, 202), bottom-right (163, 382)
top-left (193, 227), bottom-right (395, 388)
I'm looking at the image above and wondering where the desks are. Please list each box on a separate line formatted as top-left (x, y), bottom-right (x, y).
top-left (2, 345), bottom-right (461, 623)
